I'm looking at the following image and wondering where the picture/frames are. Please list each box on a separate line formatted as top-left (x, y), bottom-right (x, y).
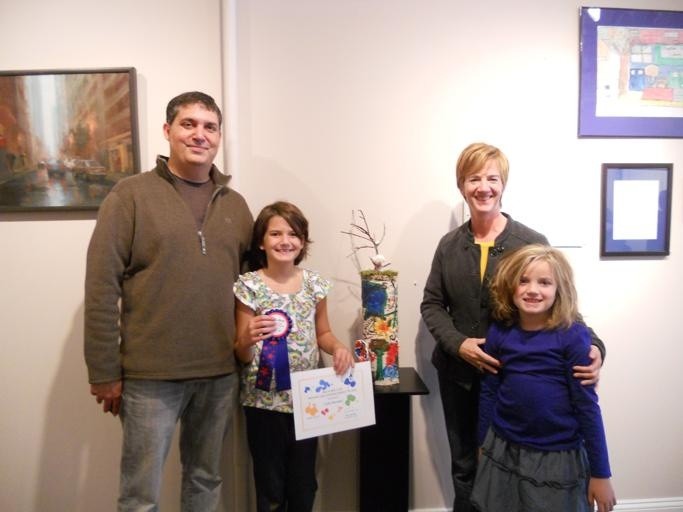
top-left (0, 67), bottom-right (140, 213)
top-left (575, 6), bottom-right (682, 140)
top-left (601, 162), bottom-right (673, 256)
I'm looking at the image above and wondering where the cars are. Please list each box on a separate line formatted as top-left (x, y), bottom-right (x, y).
top-left (36, 158), bottom-right (107, 183)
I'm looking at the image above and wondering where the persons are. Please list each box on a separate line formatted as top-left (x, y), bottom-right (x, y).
top-left (468, 244), bottom-right (617, 512)
top-left (421, 143), bottom-right (607, 512)
top-left (83, 90), bottom-right (256, 512)
top-left (235, 201), bottom-right (357, 512)
